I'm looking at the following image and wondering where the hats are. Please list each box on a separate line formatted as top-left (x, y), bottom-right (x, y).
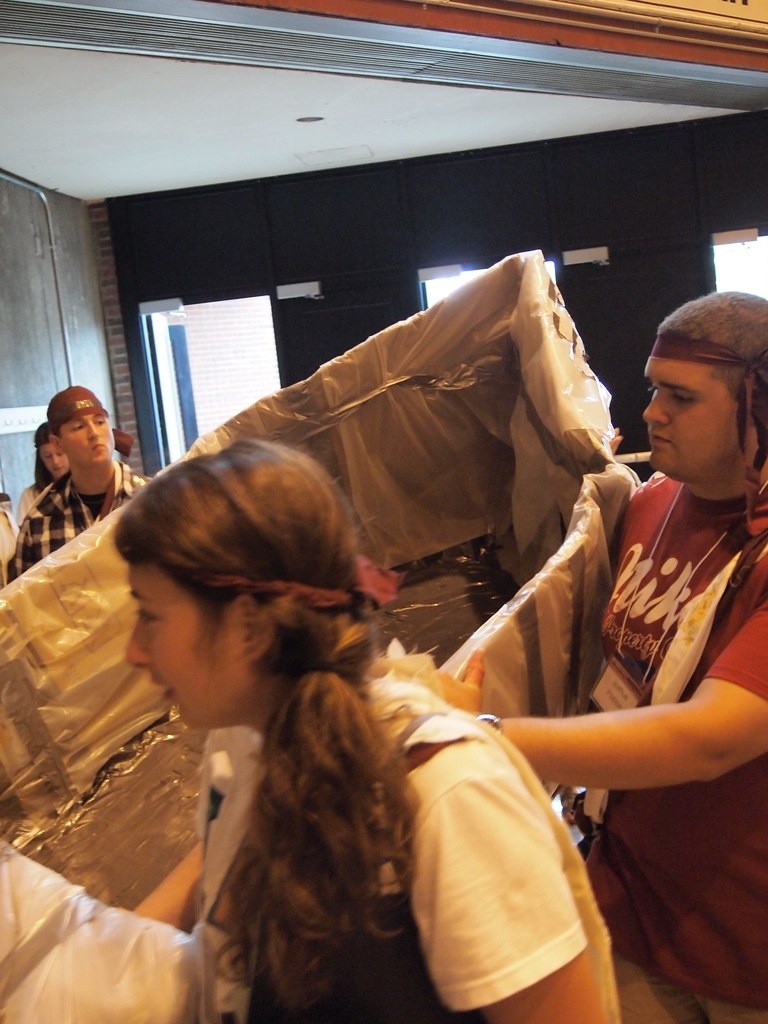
top-left (47, 386), bottom-right (109, 433)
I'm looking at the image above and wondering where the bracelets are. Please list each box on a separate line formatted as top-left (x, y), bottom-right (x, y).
top-left (478, 713), bottom-right (501, 731)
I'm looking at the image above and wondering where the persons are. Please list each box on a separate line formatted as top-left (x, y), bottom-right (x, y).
top-left (112, 438), bottom-right (625, 1024)
top-left (420, 293), bottom-right (768, 1024)
top-left (0, 385), bottom-right (152, 592)
